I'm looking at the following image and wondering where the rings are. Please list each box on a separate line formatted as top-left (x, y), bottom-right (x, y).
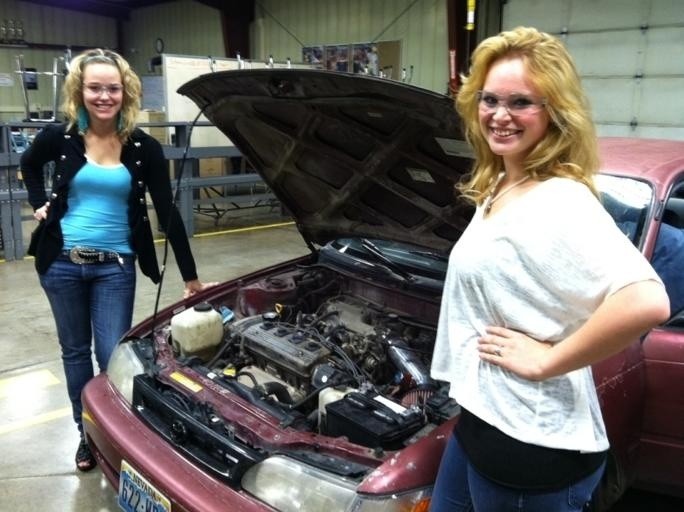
top-left (496, 348), bottom-right (502, 355)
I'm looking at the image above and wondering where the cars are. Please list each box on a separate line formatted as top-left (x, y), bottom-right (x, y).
top-left (78, 67), bottom-right (684, 512)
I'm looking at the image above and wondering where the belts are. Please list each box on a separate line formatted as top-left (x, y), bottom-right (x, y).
top-left (56, 245), bottom-right (137, 266)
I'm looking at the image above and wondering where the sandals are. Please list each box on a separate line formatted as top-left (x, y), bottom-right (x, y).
top-left (74, 437), bottom-right (98, 472)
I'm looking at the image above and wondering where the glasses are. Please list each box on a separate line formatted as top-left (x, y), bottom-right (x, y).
top-left (475, 89), bottom-right (547, 116)
top-left (82, 82), bottom-right (126, 97)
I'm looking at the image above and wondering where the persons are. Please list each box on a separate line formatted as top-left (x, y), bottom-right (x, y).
top-left (20, 48), bottom-right (222, 473)
top-left (427, 25), bottom-right (671, 512)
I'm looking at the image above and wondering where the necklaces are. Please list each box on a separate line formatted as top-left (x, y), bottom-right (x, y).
top-left (486, 173), bottom-right (532, 211)
